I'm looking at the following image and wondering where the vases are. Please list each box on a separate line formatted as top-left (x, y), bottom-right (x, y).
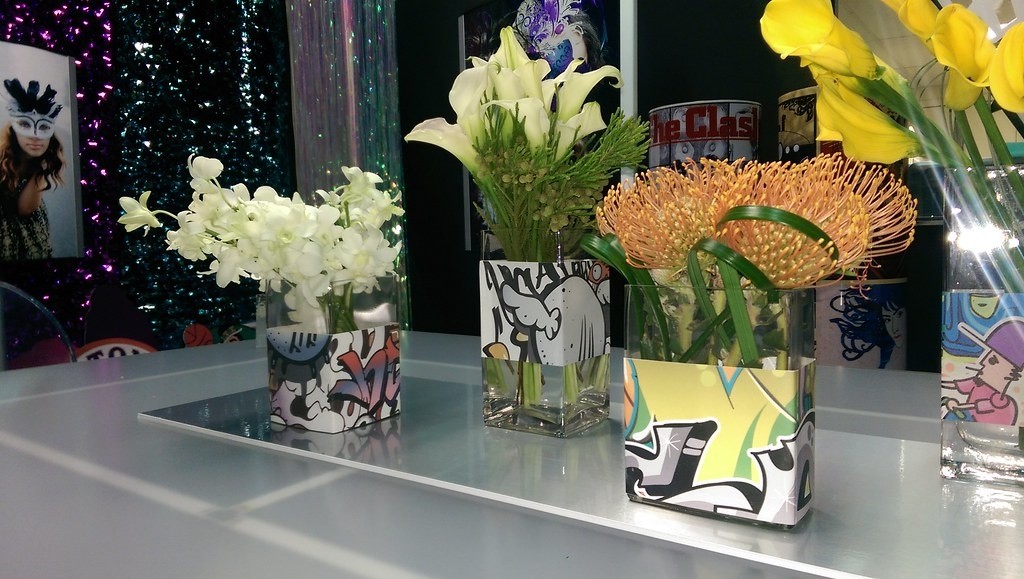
top-left (625, 285), bottom-right (822, 532)
top-left (940, 166), bottom-right (1024, 486)
top-left (265, 266), bottom-right (407, 434)
top-left (478, 231), bottom-right (612, 438)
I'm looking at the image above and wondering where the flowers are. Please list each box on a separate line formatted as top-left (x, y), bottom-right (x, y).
top-left (581, 153), bottom-right (918, 364)
top-left (405, 25), bottom-right (652, 403)
top-left (758, 1), bottom-right (1024, 295)
top-left (117, 153), bottom-right (406, 335)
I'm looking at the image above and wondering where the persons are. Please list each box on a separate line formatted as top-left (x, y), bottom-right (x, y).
top-left (0, 79), bottom-right (65, 258)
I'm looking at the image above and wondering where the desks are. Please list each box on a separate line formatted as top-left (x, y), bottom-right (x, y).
top-left (1, 328), bottom-right (1023, 578)
